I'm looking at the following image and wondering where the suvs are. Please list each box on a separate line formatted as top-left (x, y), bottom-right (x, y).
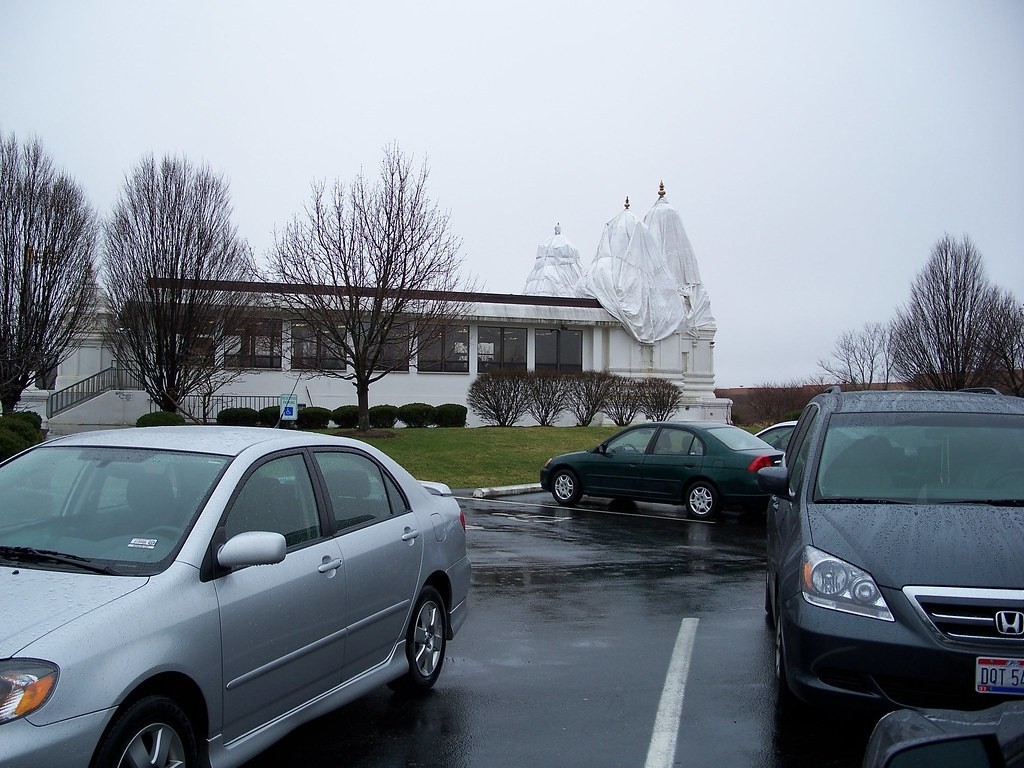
top-left (758, 386), bottom-right (1024, 710)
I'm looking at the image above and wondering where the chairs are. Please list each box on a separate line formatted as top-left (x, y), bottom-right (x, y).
top-left (655, 431), bottom-right (673, 454)
top-left (324, 470), bottom-right (382, 530)
top-left (680, 435), bottom-right (695, 453)
top-left (104, 475), bottom-right (178, 537)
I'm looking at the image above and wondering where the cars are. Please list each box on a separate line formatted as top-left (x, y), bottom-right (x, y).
top-left (541, 421), bottom-right (786, 518)
top-left (0, 423), bottom-right (471, 768)
top-left (753, 422), bottom-right (874, 463)
